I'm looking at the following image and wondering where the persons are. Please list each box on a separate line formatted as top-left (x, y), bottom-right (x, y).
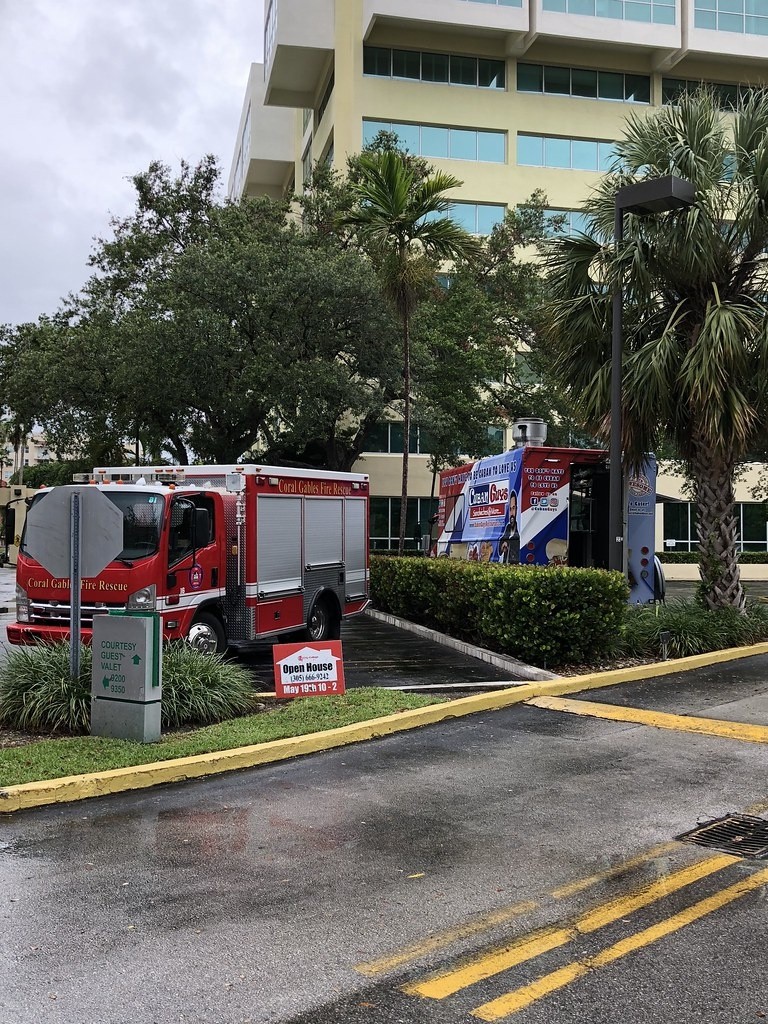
top-left (499, 489), bottom-right (520, 564)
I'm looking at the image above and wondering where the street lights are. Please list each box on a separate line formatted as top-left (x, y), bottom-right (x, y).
top-left (610, 177), bottom-right (698, 582)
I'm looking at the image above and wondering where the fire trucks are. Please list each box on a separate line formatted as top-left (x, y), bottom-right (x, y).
top-left (6, 463), bottom-right (369, 658)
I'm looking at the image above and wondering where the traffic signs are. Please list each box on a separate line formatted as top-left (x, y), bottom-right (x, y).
top-left (90, 615), bottom-right (146, 701)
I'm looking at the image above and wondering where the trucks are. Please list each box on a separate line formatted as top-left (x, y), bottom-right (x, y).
top-left (429, 447), bottom-right (656, 605)
